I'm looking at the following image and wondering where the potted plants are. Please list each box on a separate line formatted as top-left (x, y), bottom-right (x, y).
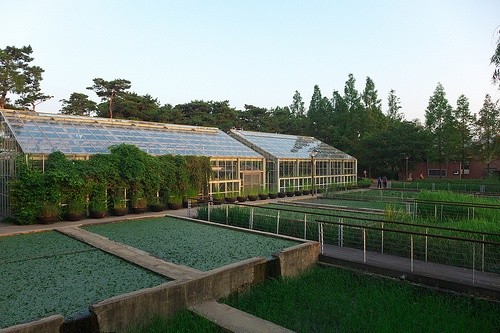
top-left (184, 188), bottom-right (198, 208)
top-left (149, 194), bottom-right (165, 212)
top-left (213, 183), bottom-right (322, 205)
top-left (88, 201), bottom-right (107, 219)
top-left (65, 199), bottom-right (82, 221)
top-left (37, 202), bottom-right (58, 225)
top-left (166, 191), bottom-right (183, 210)
top-left (131, 196), bottom-right (148, 214)
top-left (111, 197), bottom-right (129, 216)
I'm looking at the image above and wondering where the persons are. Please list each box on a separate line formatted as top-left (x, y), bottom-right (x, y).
top-left (377, 175), bottom-right (387, 188)
top-left (420, 173), bottom-right (423, 179)
